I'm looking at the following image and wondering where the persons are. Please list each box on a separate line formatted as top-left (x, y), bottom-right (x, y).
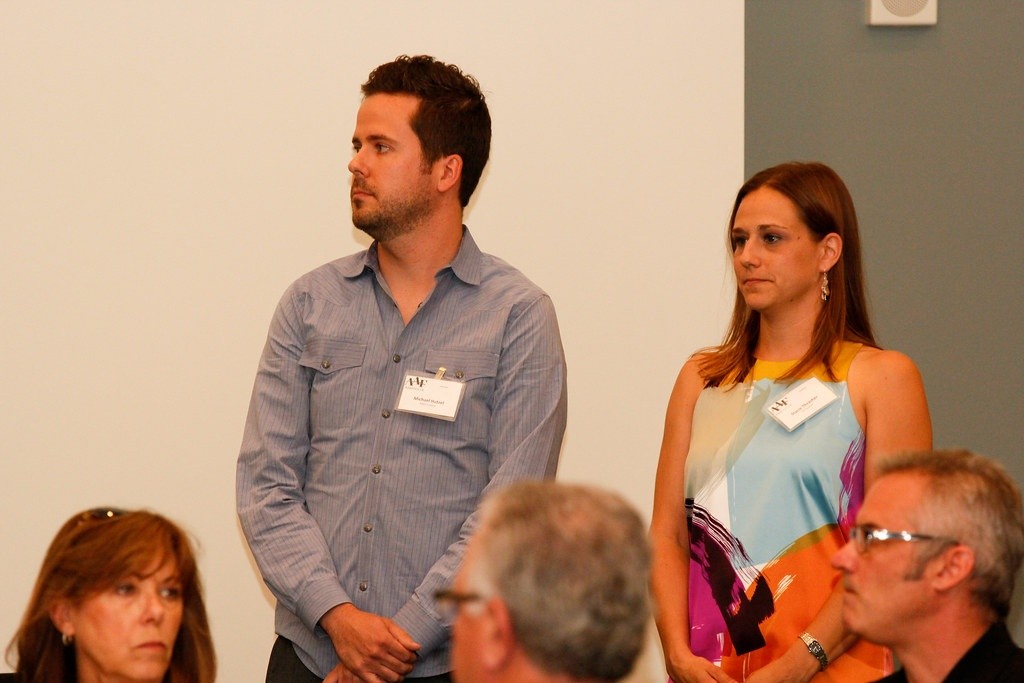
top-left (431, 480), bottom-right (660, 683)
top-left (834, 447), bottom-right (1024, 683)
top-left (234, 54), bottom-right (572, 683)
top-left (644, 162), bottom-right (935, 683)
top-left (0, 504), bottom-right (220, 683)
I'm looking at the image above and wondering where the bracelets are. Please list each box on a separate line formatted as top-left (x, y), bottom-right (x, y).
top-left (796, 629), bottom-right (829, 671)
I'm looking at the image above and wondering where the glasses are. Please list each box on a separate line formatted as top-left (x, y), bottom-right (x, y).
top-left (53, 508), bottom-right (127, 576)
top-left (849, 526), bottom-right (943, 554)
